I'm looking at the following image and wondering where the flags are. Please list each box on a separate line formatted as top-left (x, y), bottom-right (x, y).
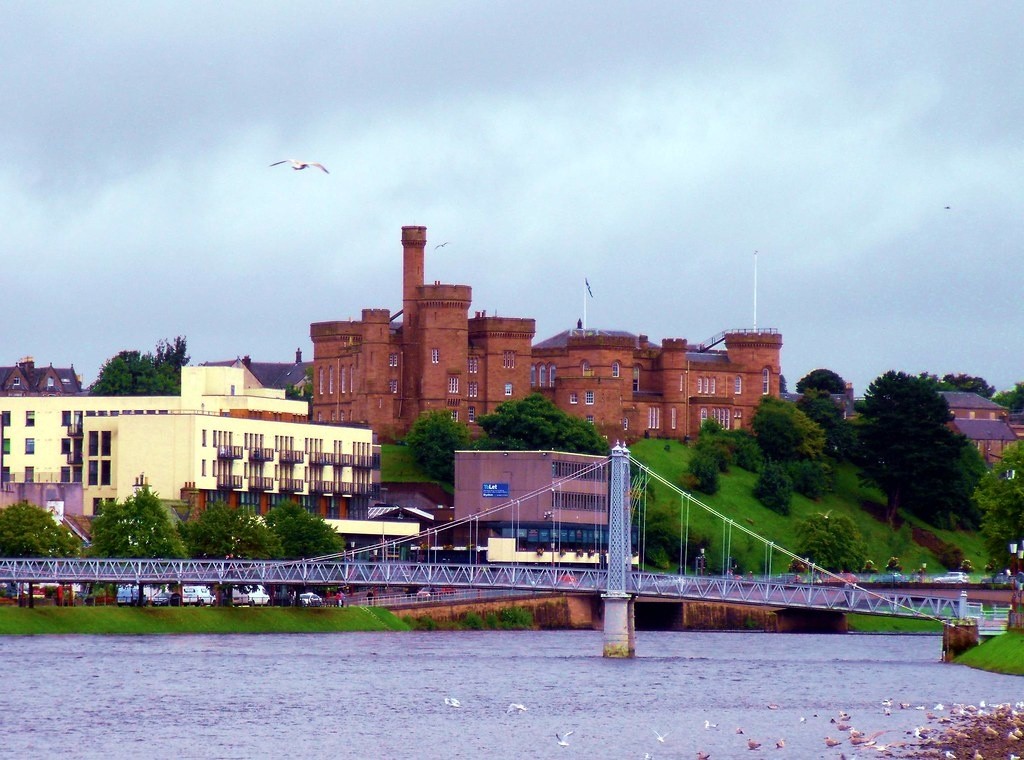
top-left (585, 278), bottom-right (593, 298)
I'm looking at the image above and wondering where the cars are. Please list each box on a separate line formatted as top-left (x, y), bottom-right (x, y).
top-left (116, 582), bottom-right (180, 606)
top-left (417, 586), bottom-right (437, 601)
top-left (934, 571), bottom-right (969, 585)
top-left (298, 593), bottom-right (323, 606)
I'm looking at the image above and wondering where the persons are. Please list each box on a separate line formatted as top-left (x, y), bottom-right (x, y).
top-left (366, 589), bottom-right (373, 605)
top-left (325, 590), bottom-right (331, 607)
top-left (334, 591), bottom-right (346, 608)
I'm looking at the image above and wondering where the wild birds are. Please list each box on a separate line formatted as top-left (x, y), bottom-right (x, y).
top-left (648, 727), bottom-right (673, 744)
top-left (268, 159), bottom-right (330, 175)
top-left (641, 752), bottom-right (656, 760)
top-left (556, 730), bottom-right (575, 748)
top-left (444, 696), bottom-right (462, 708)
top-left (505, 703), bottom-right (529, 715)
top-left (696, 751), bottom-right (711, 760)
top-left (735, 697), bottom-right (1024, 760)
top-left (703, 720), bottom-right (719, 728)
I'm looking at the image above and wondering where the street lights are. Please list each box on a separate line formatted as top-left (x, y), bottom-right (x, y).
top-left (1009, 540), bottom-right (1024, 614)
top-left (700, 547), bottom-right (705, 577)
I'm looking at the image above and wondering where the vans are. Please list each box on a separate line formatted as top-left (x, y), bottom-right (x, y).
top-left (232, 584), bottom-right (271, 607)
top-left (179, 585), bottom-right (217, 607)
